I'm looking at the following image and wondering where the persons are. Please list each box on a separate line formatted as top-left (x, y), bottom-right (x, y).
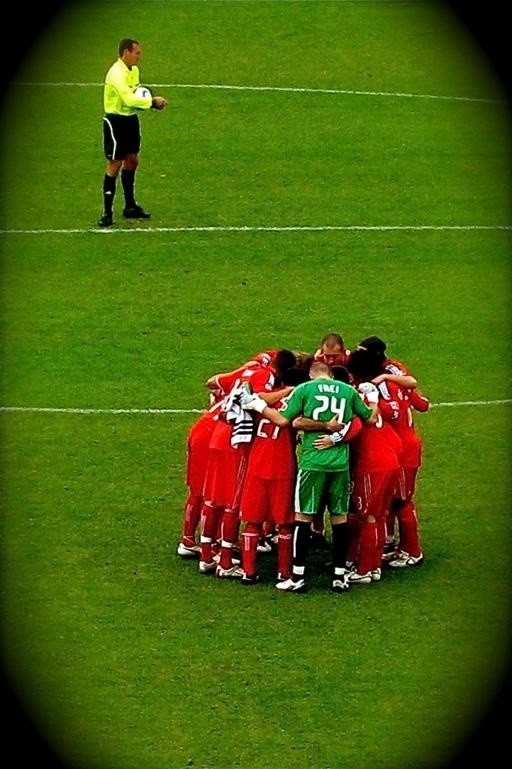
top-left (97, 37), bottom-right (169, 227)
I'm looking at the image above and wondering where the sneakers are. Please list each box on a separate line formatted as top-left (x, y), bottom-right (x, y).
top-left (96, 211), bottom-right (112, 227)
top-left (122, 205), bottom-right (151, 219)
top-left (175, 524), bottom-right (423, 592)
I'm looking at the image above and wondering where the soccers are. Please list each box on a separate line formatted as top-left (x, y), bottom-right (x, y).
top-left (131, 87), bottom-right (151, 110)
